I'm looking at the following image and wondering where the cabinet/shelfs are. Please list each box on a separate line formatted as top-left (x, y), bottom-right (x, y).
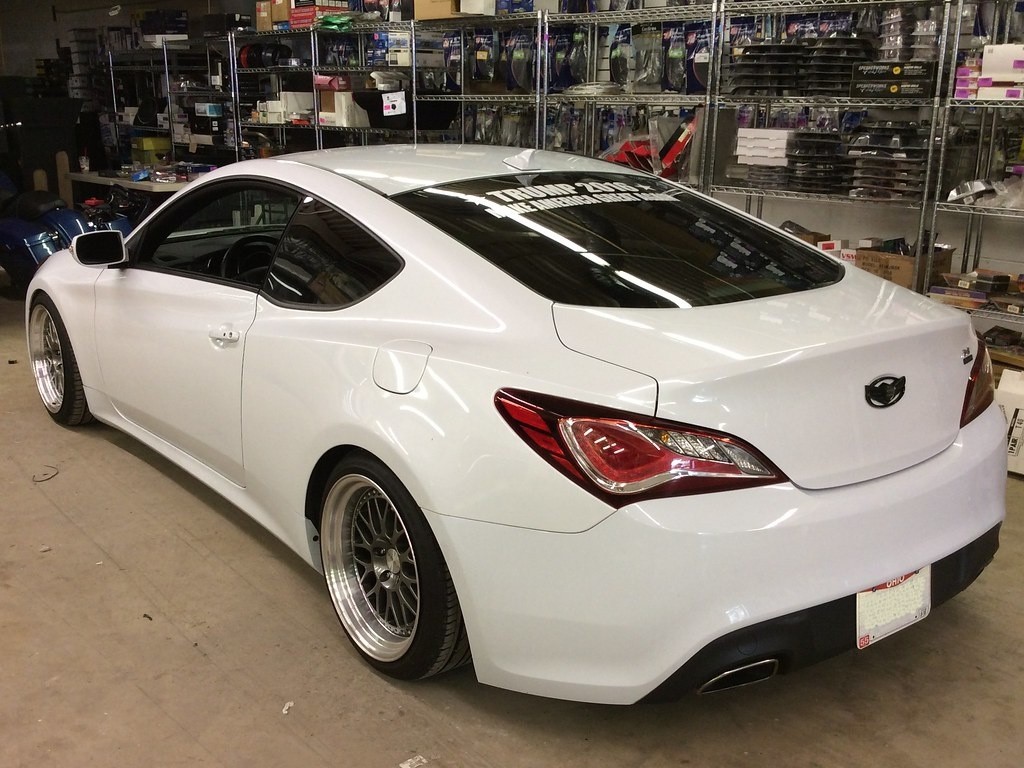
top-left (108, 37), bottom-right (173, 161)
top-left (923, 0), bottom-right (1024, 369)
top-left (314, 9), bottom-right (591, 168)
top-left (164, 30), bottom-right (238, 163)
top-left (708, 1), bottom-right (1000, 293)
top-left (544, 1), bottom-right (766, 224)
top-left (231, 23), bottom-right (320, 150)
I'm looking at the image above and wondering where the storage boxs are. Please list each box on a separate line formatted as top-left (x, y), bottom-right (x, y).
top-left (71, 53), bottom-right (89, 64)
top-left (736, 127), bottom-right (790, 167)
top-left (194, 103), bottom-right (222, 117)
top-left (851, 60), bottom-right (937, 81)
top-left (270, 0), bottom-right (349, 31)
top-left (362, 30), bottom-right (445, 66)
top-left (140, 21), bottom-right (188, 34)
top-left (124, 106), bottom-right (139, 115)
top-left (204, 13), bottom-right (251, 31)
top-left (157, 113), bottom-right (188, 129)
top-left (558, 0), bottom-right (597, 14)
top-left (644, 0), bottom-right (685, 7)
top-left (995, 369), bottom-right (1024, 477)
top-left (778, 220), bottom-right (1022, 350)
top-left (130, 137), bottom-right (171, 166)
top-left (257, 112), bottom-right (285, 124)
top-left (144, 9), bottom-right (189, 21)
top-left (319, 113), bottom-right (335, 127)
top-left (496, 0), bottom-right (533, 15)
top-left (414, 0), bottom-right (456, 19)
top-left (335, 92), bottom-right (370, 128)
top-left (69, 89), bottom-right (88, 99)
top-left (460, 0), bottom-right (496, 16)
top-left (953, 43), bottom-right (1023, 102)
top-left (73, 64), bottom-right (89, 75)
top-left (319, 91), bottom-right (335, 112)
top-left (177, 163), bottom-right (217, 182)
top-left (848, 81), bottom-right (933, 99)
top-left (314, 74), bottom-right (351, 91)
top-left (67, 28), bottom-right (96, 41)
top-left (70, 41), bottom-right (98, 53)
top-left (256, 1), bottom-right (272, 32)
top-left (115, 113), bottom-right (135, 125)
top-left (69, 75), bottom-right (88, 89)
top-left (257, 100), bottom-right (285, 111)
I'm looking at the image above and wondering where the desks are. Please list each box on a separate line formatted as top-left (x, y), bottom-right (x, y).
top-left (66, 171), bottom-right (190, 193)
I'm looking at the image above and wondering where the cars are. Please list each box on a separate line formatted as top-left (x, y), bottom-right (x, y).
top-left (26, 144), bottom-right (1012, 706)
